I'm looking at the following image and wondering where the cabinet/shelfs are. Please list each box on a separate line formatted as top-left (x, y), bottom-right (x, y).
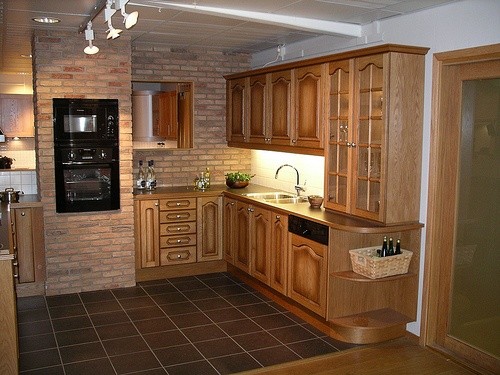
top-left (153, 92), bottom-right (193, 149)
top-left (326, 44), bottom-right (430, 225)
top-left (271, 211), bottom-right (288, 308)
top-left (9, 208), bottom-right (47, 297)
top-left (222, 196), bottom-right (235, 277)
top-left (236, 201), bottom-right (272, 298)
top-left (246, 62), bottom-right (293, 152)
top-left (330, 227), bottom-right (422, 345)
top-left (134, 200), bottom-right (160, 282)
top-left (293, 54), bottom-right (326, 156)
top-left (0, 94), bottom-right (36, 171)
top-left (158, 199), bottom-right (197, 278)
top-left (223, 72), bottom-right (246, 148)
top-left (196, 196), bottom-right (227, 275)
top-left (286, 231), bottom-right (328, 333)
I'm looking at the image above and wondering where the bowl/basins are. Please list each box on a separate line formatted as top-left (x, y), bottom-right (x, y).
top-left (226, 179), bottom-right (248, 189)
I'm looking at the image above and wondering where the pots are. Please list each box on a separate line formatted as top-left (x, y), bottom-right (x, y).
top-left (0, 187), bottom-right (20, 203)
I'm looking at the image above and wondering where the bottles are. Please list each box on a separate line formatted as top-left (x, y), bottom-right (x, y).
top-left (395, 240), bottom-right (401, 255)
top-left (380, 236), bottom-right (388, 257)
top-left (388, 238), bottom-right (394, 256)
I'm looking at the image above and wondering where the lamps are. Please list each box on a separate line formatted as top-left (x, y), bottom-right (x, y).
top-left (104, 0), bottom-right (122, 40)
top-left (84, 20), bottom-right (99, 55)
top-left (115, 0), bottom-right (139, 30)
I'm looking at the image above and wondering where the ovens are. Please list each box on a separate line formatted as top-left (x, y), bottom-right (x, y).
top-left (52, 97), bottom-right (121, 213)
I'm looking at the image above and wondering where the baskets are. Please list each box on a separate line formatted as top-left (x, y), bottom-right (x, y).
top-left (349, 245), bottom-right (413, 279)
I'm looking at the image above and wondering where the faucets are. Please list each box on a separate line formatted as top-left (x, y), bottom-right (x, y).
top-left (276, 164), bottom-right (306, 197)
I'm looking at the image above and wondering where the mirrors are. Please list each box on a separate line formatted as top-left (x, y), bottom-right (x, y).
top-left (131, 79), bottom-right (195, 151)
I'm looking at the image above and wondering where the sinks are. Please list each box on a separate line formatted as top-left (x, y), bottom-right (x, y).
top-left (268, 197), bottom-right (307, 206)
top-left (248, 192), bottom-right (296, 200)
